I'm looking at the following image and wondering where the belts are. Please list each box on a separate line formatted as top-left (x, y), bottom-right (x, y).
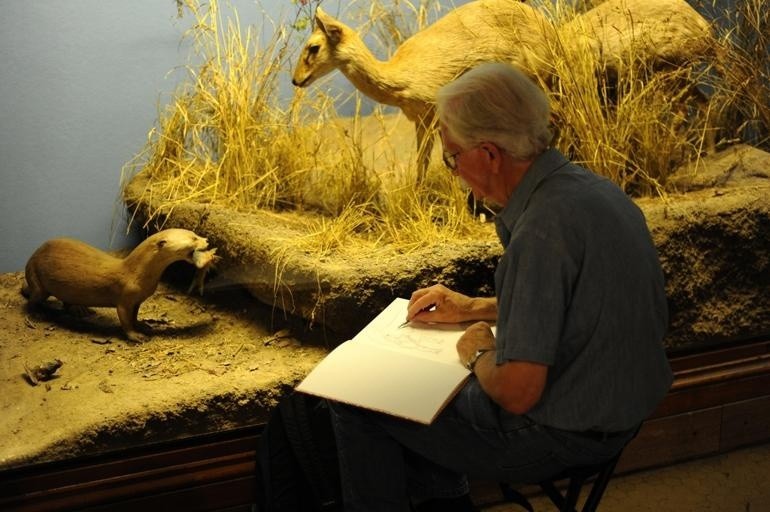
top-left (544, 421), bottom-right (641, 439)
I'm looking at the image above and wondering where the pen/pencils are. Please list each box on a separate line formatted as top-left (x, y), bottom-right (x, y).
top-left (399, 304), bottom-right (436, 329)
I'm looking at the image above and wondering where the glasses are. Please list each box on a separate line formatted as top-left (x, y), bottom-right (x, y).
top-left (442, 141), bottom-right (507, 171)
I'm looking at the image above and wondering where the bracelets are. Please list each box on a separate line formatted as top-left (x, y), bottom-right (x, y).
top-left (466, 347), bottom-right (495, 373)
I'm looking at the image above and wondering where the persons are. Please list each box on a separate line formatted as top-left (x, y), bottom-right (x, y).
top-left (328, 60), bottom-right (675, 510)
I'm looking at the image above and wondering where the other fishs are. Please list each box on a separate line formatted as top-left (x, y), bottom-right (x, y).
top-left (187, 247), bottom-right (224, 296)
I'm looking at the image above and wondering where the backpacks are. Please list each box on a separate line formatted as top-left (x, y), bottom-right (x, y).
top-left (253, 392), bottom-right (341, 511)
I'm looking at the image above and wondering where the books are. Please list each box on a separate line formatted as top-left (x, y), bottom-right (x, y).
top-left (295, 295), bottom-right (498, 429)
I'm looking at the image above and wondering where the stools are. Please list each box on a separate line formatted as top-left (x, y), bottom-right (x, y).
top-left (498, 418), bottom-right (644, 511)
